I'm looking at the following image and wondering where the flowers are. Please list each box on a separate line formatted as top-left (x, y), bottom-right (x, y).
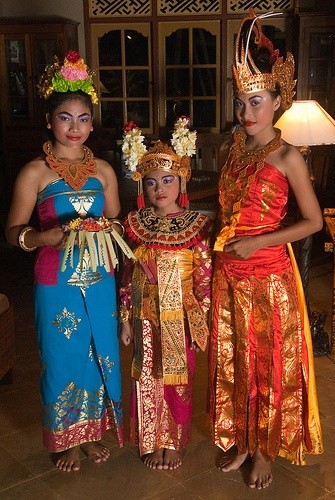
top-left (52, 50), bottom-right (101, 105)
top-left (121, 121), bottom-right (147, 171)
top-left (170, 116), bottom-right (197, 157)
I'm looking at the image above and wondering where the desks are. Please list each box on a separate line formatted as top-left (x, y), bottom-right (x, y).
top-left (119, 170), bottom-right (220, 210)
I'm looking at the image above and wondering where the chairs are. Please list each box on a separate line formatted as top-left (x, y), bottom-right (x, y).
top-left (191, 133), bottom-right (224, 172)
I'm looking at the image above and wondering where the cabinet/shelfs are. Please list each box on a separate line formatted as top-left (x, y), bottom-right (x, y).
top-left (285, 23), bottom-right (335, 114)
top-left (1, 23), bottom-right (81, 126)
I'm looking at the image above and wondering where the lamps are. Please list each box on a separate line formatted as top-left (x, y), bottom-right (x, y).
top-left (274, 99), bottom-right (335, 356)
